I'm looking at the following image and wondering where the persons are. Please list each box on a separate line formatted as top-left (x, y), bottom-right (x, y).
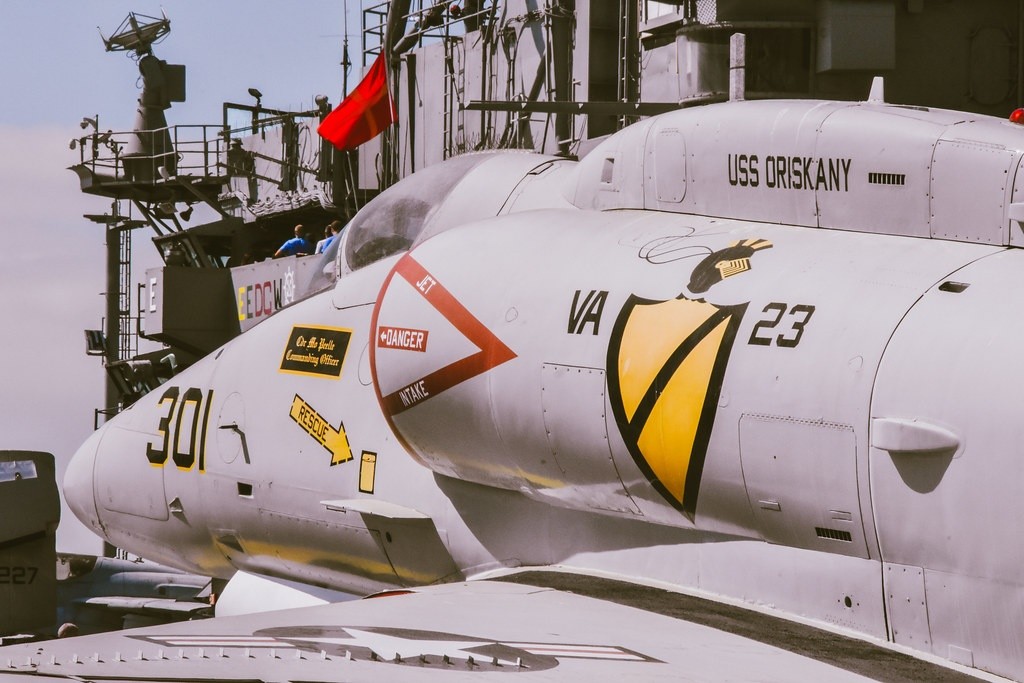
top-left (272, 224), bottom-right (315, 259)
top-left (315, 220), bottom-right (343, 255)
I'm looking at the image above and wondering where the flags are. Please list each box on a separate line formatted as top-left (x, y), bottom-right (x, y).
top-left (316, 49), bottom-right (398, 151)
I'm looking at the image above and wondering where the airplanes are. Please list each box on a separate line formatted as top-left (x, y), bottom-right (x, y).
top-left (0, 29), bottom-right (1024, 683)
